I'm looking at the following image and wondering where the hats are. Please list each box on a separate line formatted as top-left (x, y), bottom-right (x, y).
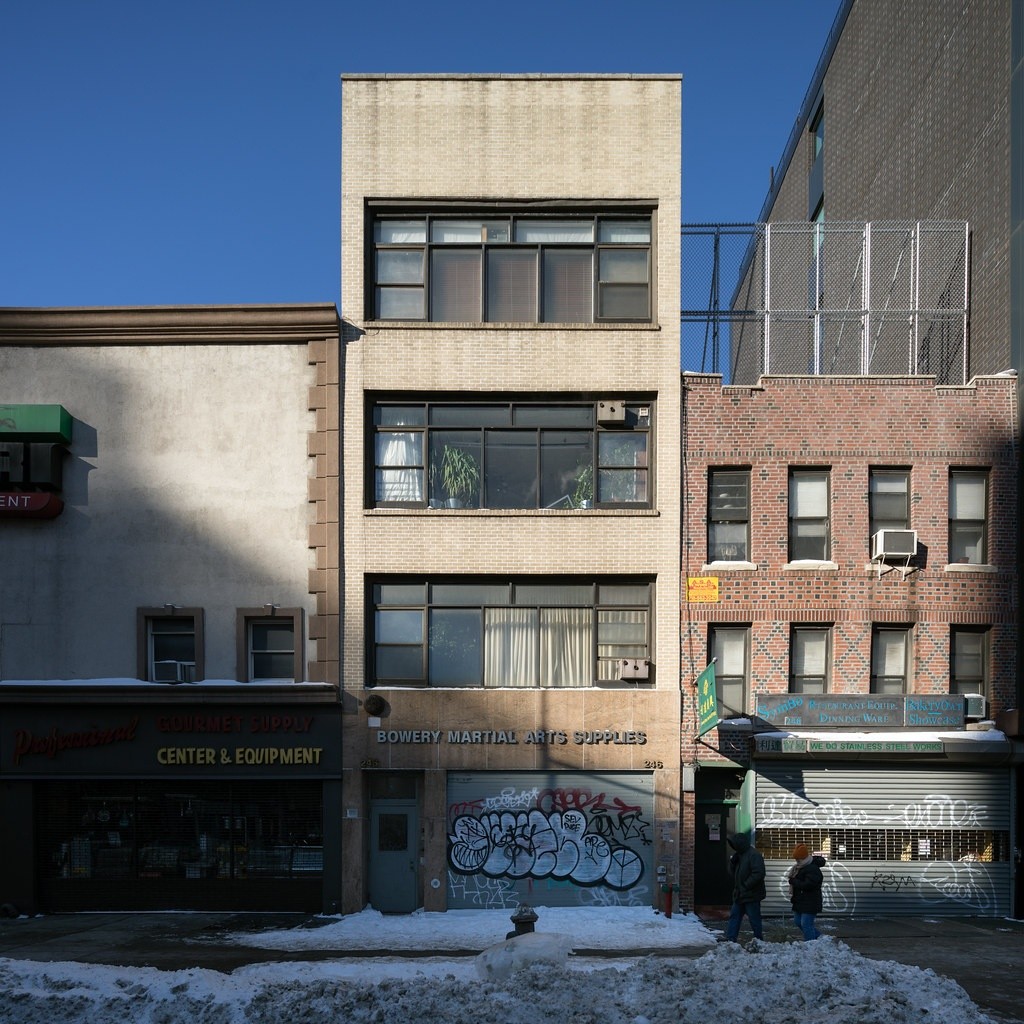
top-left (792, 844), bottom-right (808, 859)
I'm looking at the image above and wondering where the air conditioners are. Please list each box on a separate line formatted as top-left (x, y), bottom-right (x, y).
top-left (153, 660), bottom-right (193, 684)
top-left (871, 529), bottom-right (917, 559)
top-left (964, 693), bottom-right (986, 719)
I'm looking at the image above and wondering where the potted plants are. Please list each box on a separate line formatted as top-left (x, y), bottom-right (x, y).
top-left (564, 439), bottom-right (637, 509)
top-left (440, 444), bottom-right (488, 509)
top-left (429, 447), bottom-right (443, 508)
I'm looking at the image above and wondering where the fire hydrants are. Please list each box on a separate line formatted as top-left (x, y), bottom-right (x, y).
top-left (506, 902), bottom-right (539, 942)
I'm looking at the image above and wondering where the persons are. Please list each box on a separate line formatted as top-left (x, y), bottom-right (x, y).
top-left (716, 833), bottom-right (767, 944)
top-left (790, 844), bottom-right (823, 943)
